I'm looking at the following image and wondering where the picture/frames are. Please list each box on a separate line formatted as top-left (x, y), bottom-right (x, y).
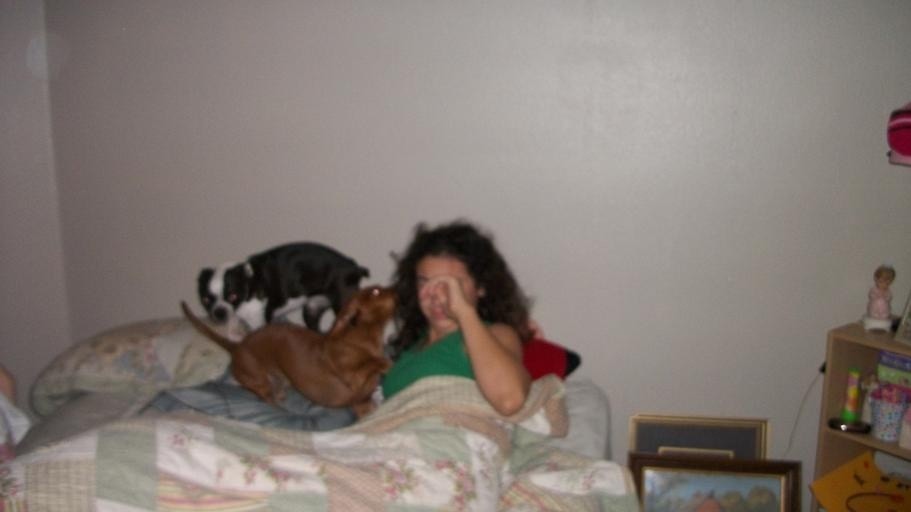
top-left (627, 452), bottom-right (801, 511)
top-left (627, 416), bottom-right (769, 463)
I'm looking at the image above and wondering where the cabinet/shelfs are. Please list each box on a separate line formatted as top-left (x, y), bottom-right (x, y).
top-left (812, 317), bottom-right (911, 509)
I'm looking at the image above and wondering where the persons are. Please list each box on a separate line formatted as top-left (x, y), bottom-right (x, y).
top-left (859, 265), bottom-right (898, 335)
top-left (345, 216), bottom-right (538, 418)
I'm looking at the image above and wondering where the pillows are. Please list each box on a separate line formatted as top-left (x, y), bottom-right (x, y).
top-left (29, 317), bottom-right (241, 392)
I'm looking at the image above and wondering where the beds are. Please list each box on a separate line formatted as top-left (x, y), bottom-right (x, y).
top-left (1, 364), bottom-right (638, 510)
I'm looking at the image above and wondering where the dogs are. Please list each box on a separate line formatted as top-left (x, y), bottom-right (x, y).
top-left (197, 242), bottom-right (370, 332)
top-left (180, 285), bottom-right (405, 422)
